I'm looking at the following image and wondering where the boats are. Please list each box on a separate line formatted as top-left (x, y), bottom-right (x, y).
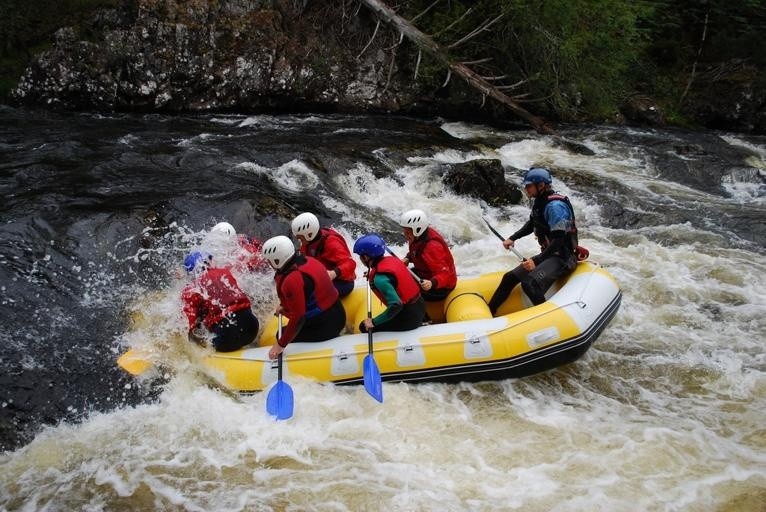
top-left (118, 258), bottom-right (622, 392)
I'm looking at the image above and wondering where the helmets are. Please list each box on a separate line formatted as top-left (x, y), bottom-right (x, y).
top-left (522, 168), bottom-right (551, 184)
top-left (182, 209), bottom-right (430, 277)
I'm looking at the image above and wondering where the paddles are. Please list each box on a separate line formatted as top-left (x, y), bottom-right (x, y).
top-left (363, 271), bottom-right (383, 404)
top-left (266, 312), bottom-right (294, 419)
top-left (481, 217), bottom-right (528, 262)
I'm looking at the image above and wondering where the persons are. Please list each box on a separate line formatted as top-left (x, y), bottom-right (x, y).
top-left (179, 251), bottom-right (259, 352)
top-left (291, 212), bottom-right (357, 300)
top-left (352, 235), bottom-right (426, 332)
top-left (210, 220), bottom-right (272, 274)
top-left (261, 235), bottom-right (346, 360)
top-left (399, 209), bottom-right (457, 326)
top-left (487, 169), bottom-right (577, 315)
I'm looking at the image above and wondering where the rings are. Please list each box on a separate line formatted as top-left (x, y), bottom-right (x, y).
top-left (273, 353), bottom-right (278, 356)
